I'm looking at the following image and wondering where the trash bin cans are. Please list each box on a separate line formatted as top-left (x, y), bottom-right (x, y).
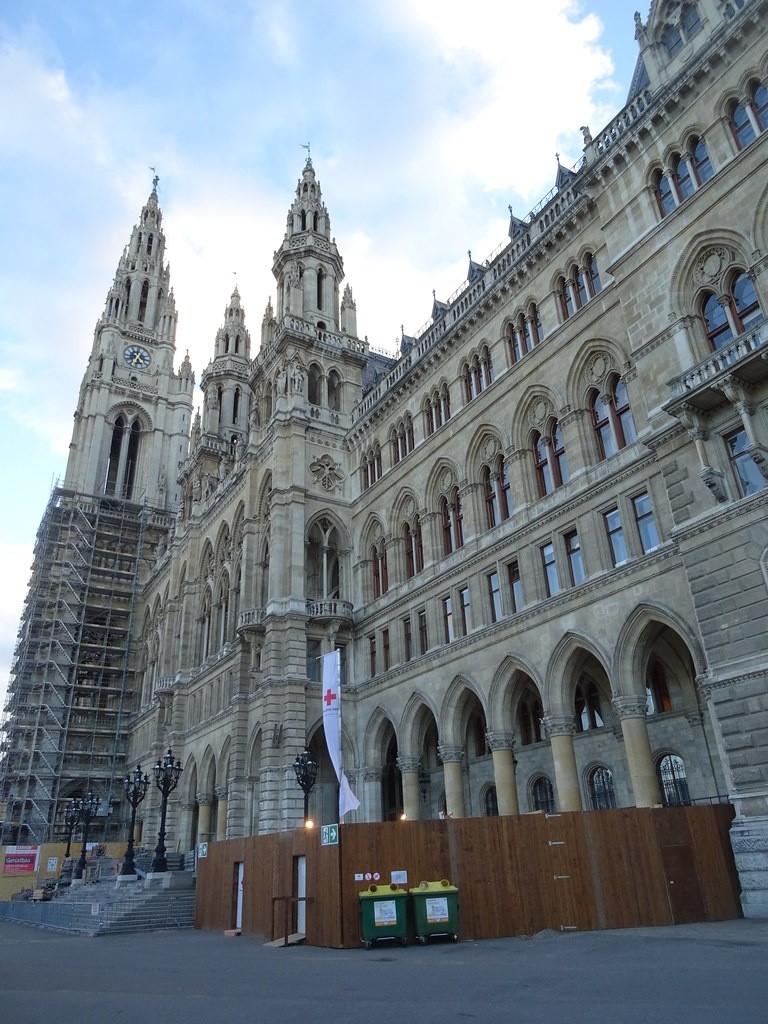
top-left (358, 882), bottom-right (410, 950)
top-left (409, 880), bottom-right (460, 947)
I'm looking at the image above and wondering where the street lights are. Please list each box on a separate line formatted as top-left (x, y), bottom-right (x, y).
top-left (293, 743), bottom-right (322, 827)
top-left (63, 797), bottom-right (82, 858)
top-left (149, 745), bottom-right (185, 872)
top-left (80, 792), bottom-right (101, 869)
top-left (118, 760), bottom-right (152, 875)
